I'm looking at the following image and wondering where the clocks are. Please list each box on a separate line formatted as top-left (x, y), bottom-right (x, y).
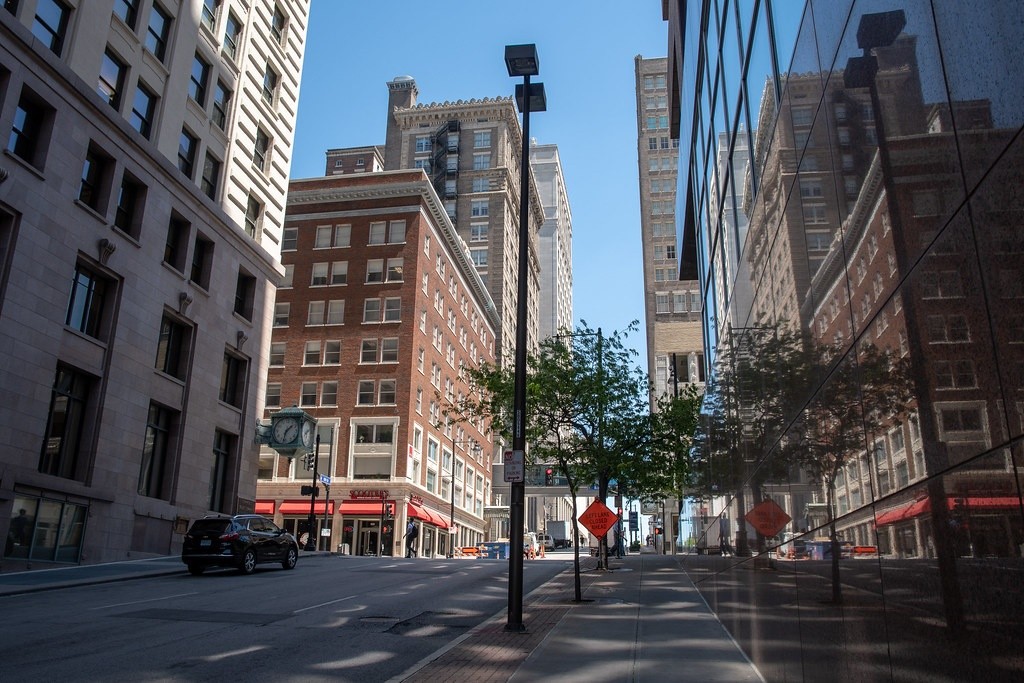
top-left (301, 419), bottom-right (314, 448)
top-left (273, 417), bottom-right (298, 444)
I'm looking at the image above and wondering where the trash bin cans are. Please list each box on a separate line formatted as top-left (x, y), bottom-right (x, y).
top-left (337, 543), bottom-right (349, 555)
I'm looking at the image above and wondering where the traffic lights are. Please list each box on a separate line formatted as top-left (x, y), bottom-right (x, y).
top-left (385, 504), bottom-right (392, 520)
top-left (545, 467), bottom-right (554, 486)
top-left (656, 528), bottom-right (662, 535)
top-left (304, 452), bottom-right (314, 471)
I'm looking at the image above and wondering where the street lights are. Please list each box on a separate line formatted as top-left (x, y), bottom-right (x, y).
top-left (450, 439), bottom-right (482, 557)
top-left (504, 42), bottom-right (547, 632)
top-left (552, 327), bottom-right (608, 570)
top-left (844, 8), bottom-right (974, 683)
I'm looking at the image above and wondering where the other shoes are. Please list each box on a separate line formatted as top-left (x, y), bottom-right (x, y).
top-left (405, 555), bottom-right (411, 558)
top-left (415, 551), bottom-right (416, 558)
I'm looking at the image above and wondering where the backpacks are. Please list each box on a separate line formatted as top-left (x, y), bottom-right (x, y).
top-left (408, 523), bottom-right (418, 538)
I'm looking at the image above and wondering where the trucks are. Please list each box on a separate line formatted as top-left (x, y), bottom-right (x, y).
top-left (546, 520), bottom-right (573, 549)
top-left (587, 520), bottom-right (626, 557)
top-left (690, 515), bottom-right (729, 554)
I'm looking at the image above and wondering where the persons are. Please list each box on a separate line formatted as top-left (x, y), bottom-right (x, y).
top-left (403, 518), bottom-right (418, 559)
top-left (580, 535), bottom-right (584, 548)
top-left (609, 514), bottom-right (623, 556)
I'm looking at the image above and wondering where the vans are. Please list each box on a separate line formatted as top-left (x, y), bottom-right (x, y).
top-left (779, 532), bottom-right (809, 556)
top-left (537, 534), bottom-right (555, 552)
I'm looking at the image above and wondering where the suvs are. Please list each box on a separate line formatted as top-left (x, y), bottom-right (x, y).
top-left (182, 514), bottom-right (298, 575)
